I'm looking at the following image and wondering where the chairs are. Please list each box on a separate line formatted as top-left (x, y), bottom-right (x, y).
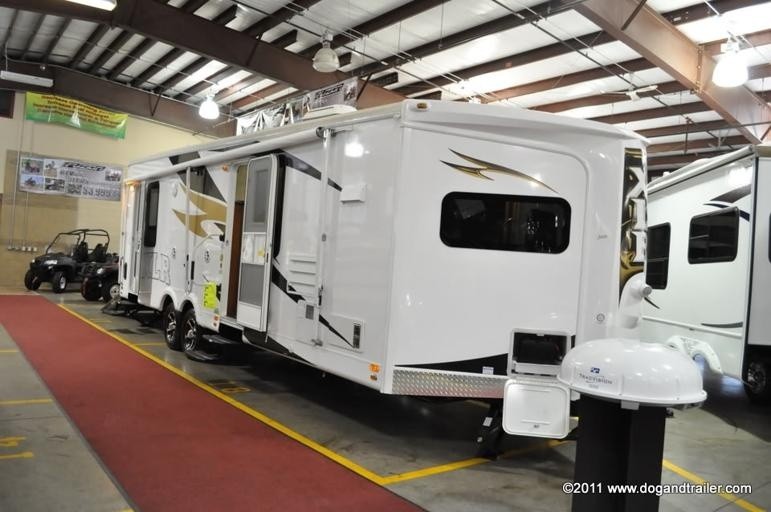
top-left (73, 241), bottom-right (102, 262)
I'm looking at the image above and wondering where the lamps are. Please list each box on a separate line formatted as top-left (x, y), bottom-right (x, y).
top-left (310, 33), bottom-right (340, 72)
top-left (711, 36), bottom-right (748, 88)
